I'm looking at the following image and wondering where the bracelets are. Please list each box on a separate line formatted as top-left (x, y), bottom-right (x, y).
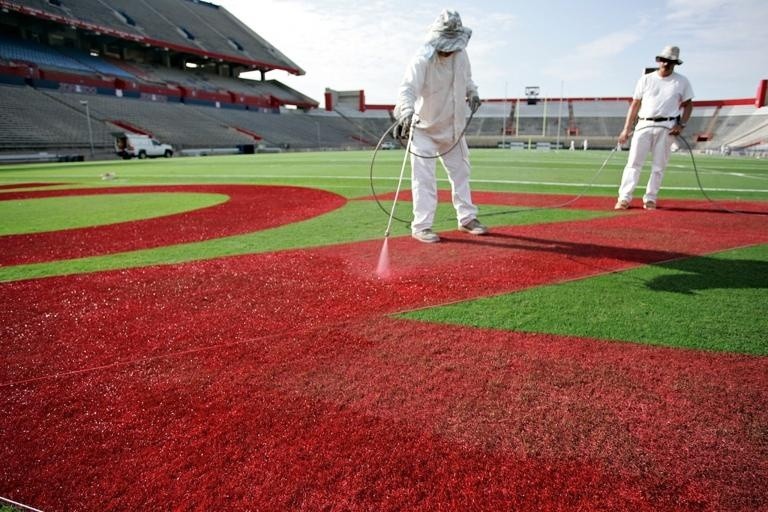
top-left (677, 120), bottom-right (687, 128)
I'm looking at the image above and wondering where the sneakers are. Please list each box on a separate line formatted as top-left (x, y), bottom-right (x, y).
top-left (615, 198), bottom-right (632, 210)
top-left (458, 218), bottom-right (488, 235)
top-left (643, 200), bottom-right (658, 212)
top-left (411, 228), bottom-right (440, 244)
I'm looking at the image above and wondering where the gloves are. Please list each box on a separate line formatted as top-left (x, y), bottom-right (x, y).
top-left (469, 96), bottom-right (480, 113)
top-left (393, 121), bottom-right (407, 140)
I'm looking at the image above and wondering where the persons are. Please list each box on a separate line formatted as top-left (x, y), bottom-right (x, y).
top-left (613, 45), bottom-right (695, 212)
top-left (391, 9), bottom-right (488, 245)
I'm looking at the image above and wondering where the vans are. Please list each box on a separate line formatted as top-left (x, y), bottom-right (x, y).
top-left (110, 131), bottom-right (175, 160)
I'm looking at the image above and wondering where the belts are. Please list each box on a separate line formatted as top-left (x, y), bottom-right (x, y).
top-left (638, 116), bottom-right (677, 122)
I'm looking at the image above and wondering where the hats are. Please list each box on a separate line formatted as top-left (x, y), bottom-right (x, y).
top-left (656, 45), bottom-right (683, 66)
top-left (422, 8), bottom-right (473, 54)
